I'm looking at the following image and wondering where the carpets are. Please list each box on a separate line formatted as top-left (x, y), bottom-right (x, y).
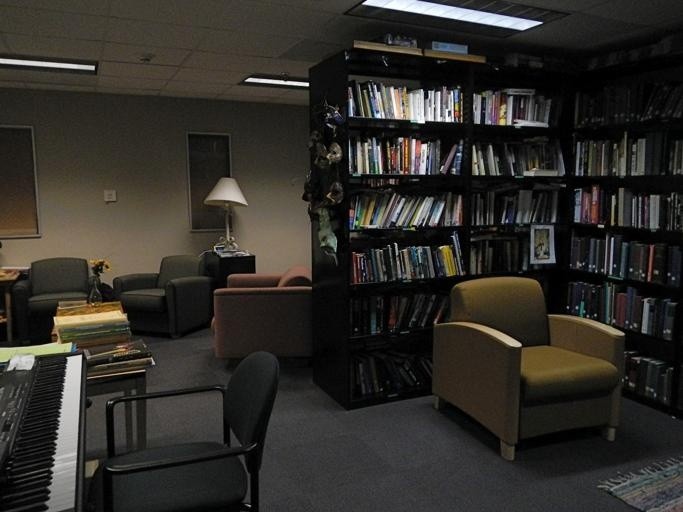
top-left (598, 457), bottom-right (683, 510)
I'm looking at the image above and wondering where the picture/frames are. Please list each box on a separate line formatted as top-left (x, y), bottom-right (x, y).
top-left (186, 132), bottom-right (235, 234)
top-left (0, 125), bottom-right (41, 240)
top-left (529, 223), bottom-right (556, 265)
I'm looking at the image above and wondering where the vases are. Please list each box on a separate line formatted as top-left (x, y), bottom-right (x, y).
top-left (89, 277), bottom-right (103, 303)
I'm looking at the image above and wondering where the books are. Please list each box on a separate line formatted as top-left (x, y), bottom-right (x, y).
top-left (507, 53), bottom-right (544, 69)
top-left (468, 87), bottom-right (566, 276)
top-left (347, 79), bottom-right (465, 396)
top-left (353, 33), bottom-right (486, 64)
top-left (51, 301), bottom-right (156, 377)
top-left (565, 78), bottom-right (683, 405)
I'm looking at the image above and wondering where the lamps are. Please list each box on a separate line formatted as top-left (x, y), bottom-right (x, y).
top-left (204, 176), bottom-right (248, 252)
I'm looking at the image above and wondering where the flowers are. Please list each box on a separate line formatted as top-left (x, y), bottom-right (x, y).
top-left (88, 258), bottom-right (112, 278)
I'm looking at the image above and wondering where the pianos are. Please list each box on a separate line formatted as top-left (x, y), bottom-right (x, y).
top-left (0, 351), bottom-right (88, 512)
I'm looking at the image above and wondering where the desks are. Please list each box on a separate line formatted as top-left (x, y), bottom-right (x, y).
top-left (0, 271), bottom-right (23, 342)
top-left (87, 369), bottom-right (147, 451)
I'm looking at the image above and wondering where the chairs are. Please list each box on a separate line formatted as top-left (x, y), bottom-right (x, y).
top-left (210, 264), bottom-right (313, 368)
top-left (87, 351), bottom-right (279, 508)
top-left (113, 255), bottom-right (213, 339)
top-left (434, 276), bottom-right (626, 463)
top-left (12, 258), bottom-right (111, 344)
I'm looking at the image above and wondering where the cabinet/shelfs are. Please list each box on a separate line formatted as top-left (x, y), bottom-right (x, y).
top-left (206, 252), bottom-right (256, 288)
top-left (307, 40), bottom-right (681, 420)
